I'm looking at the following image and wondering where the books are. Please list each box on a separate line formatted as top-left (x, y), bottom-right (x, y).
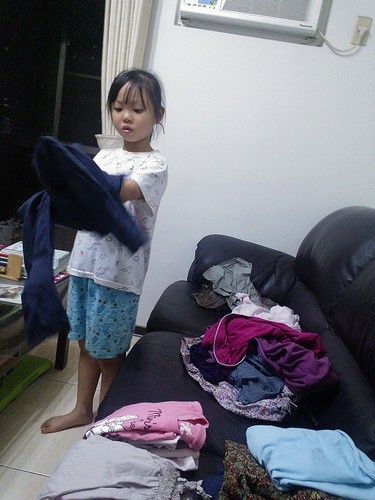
top-left (0, 240), bottom-right (71, 279)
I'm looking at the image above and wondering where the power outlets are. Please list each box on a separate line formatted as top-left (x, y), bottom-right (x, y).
top-left (351, 15), bottom-right (373, 46)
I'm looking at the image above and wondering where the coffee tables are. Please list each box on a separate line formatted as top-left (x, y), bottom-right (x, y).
top-left (0, 270), bottom-right (72, 370)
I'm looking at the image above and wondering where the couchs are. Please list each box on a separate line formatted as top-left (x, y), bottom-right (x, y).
top-left (95, 206), bottom-right (375, 482)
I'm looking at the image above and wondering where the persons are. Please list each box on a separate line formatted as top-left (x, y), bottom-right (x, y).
top-left (18, 68), bottom-right (169, 434)
